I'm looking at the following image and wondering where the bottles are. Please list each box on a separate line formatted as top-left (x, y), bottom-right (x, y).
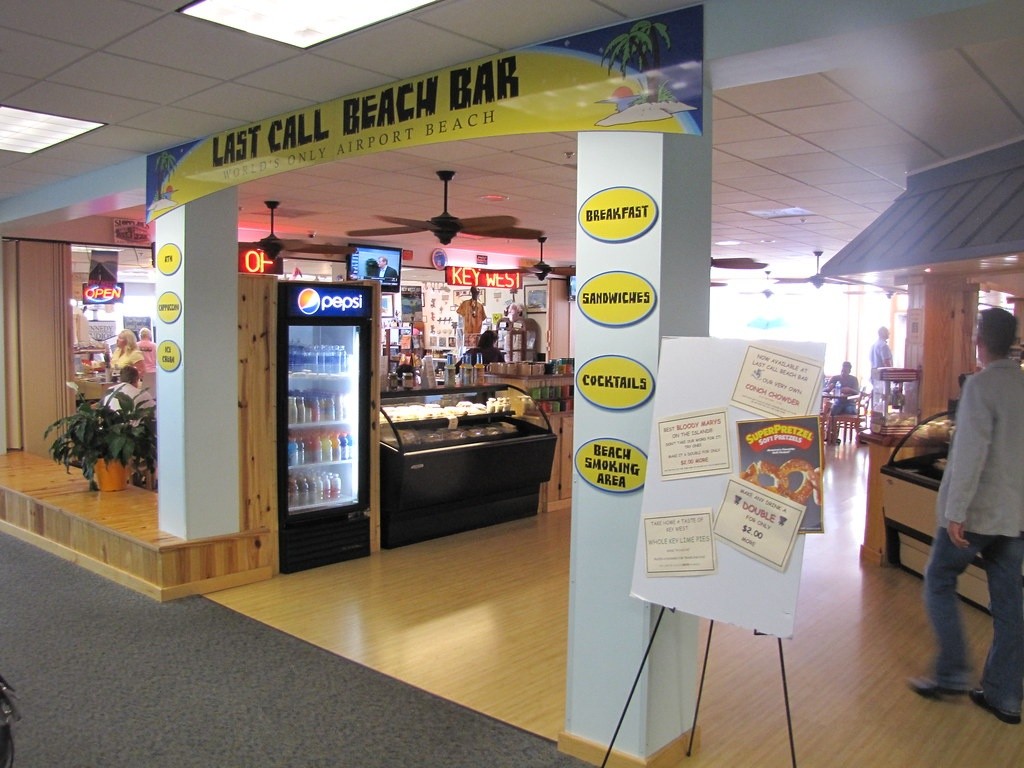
top-left (474, 353), bottom-right (485, 385)
top-left (486, 397), bottom-right (510, 413)
top-left (459, 356), bottom-right (466, 385)
top-left (288, 469), bottom-right (342, 508)
top-left (288, 429), bottom-right (354, 464)
top-left (289, 337), bottom-right (347, 374)
top-left (463, 354), bottom-right (473, 385)
top-left (444, 354), bottom-right (458, 387)
top-left (288, 387), bottom-right (346, 424)
top-left (836, 381), bottom-right (841, 395)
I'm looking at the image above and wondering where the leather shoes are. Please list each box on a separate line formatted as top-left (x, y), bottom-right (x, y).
top-left (906, 677), bottom-right (967, 698)
top-left (969, 687), bottom-right (1022, 724)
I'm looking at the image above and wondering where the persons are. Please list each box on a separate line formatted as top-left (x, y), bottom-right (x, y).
top-left (908, 306), bottom-right (1023, 724)
top-left (375, 256), bottom-right (397, 277)
top-left (109, 329), bottom-right (146, 385)
top-left (825, 362), bottom-right (865, 443)
top-left (103, 366), bottom-right (157, 426)
top-left (455, 330), bottom-right (505, 374)
top-left (397, 365), bottom-right (417, 388)
top-left (137, 327), bottom-right (156, 368)
top-left (871, 326), bottom-right (893, 408)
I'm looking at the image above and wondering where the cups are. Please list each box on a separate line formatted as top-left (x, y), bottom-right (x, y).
top-left (486, 357), bottom-right (574, 376)
top-left (528, 386), bottom-right (572, 413)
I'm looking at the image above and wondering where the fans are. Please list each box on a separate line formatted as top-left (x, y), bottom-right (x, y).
top-left (772, 249), bottom-right (857, 292)
top-left (489, 235), bottom-right (576, 285)
top-left (707, 281), bottom-right (731, 290)
top-left (738, 270), bottom-right (800, 298)
top-left (250, 201), bottom-right (356, 269)
top-left (710, 253), bottom-right (767, 277)
top-left (345, 169), bottom-right (548, 247)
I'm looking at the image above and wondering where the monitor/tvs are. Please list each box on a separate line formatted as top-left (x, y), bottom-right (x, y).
top-left (346, 242), bottom-right (406, 292)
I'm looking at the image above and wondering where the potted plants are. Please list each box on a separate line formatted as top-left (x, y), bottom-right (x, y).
top-left (39, 382), bottom-right (159, 496)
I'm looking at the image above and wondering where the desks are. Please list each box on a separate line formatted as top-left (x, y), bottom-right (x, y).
top-left (810, 393), bottom-right (849, 448)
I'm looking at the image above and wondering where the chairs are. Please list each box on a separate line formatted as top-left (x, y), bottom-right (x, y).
top-left (826, 389), bottom-right (873, 446)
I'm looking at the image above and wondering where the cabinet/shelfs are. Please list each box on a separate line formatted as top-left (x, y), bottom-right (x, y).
top-left (478, 354), bottom-right (574, 416)
top-left (533, 412), bottom-right (573, 510)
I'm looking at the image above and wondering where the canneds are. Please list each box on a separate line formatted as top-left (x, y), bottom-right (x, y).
top-left (531, 385), bottom-right (570, 415)
top-left (549, 357), bottom-right (573, 375)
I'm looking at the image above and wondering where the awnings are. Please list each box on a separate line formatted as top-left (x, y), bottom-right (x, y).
top-left (821, 168), bottom-right (1024, 276)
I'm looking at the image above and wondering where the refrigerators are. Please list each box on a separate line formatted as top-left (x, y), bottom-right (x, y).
top-left (277, 278), bottom-right (371, 573)
